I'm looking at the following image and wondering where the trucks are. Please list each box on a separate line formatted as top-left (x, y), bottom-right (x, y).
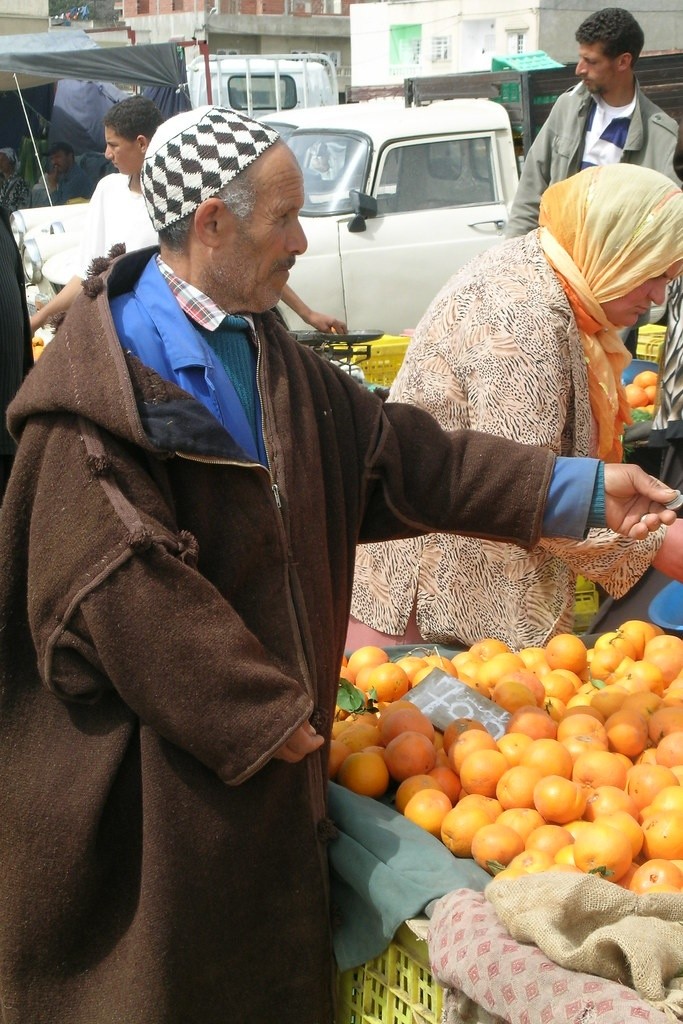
top-left (184, 53), bottom-right (338, 123)
top-left (275, 53), bottom-right (683, 341)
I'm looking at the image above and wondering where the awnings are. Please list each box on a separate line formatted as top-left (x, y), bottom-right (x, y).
top-left (1, 29), bottom-right (190, 106)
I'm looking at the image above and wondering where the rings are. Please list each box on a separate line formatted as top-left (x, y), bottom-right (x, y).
top-left (640, 512), bottom-right (650, 521)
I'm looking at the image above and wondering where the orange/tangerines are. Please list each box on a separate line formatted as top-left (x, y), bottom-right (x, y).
top-left (31, 336), bottom-right (45, 362)
top-left (625, 370), bottom-right (657, 415)
top-left (324, 621), bottom-right (683, 898)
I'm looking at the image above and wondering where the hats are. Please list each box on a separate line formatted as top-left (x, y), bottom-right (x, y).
top-left (41, 142), bottom-right (73, 156)
top-left (139, 104), bottom-right (279, 232)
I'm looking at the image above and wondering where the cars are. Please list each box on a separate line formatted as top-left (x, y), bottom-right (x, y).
top-left (9, 73), bottom-right (400, 309)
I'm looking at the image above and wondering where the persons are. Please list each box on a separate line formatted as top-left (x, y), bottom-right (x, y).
top-left (342, 163), bottom-right (683, 649)
top-left (0, 146), bottom-right (32, 211)
top-left (30, 94), bottom-right (165, 335)
top-left (43, 142), bottom-right (93, 206)
top-left (503, 9), bottom-right (683, 238)
top-left (0, 106), bottom-right (678, 1024)
top-left (0, 224), bottom-right (39, 427)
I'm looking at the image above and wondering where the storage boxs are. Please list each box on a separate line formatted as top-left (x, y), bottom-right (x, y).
top-left (329, 922), bottom-right (445, 1024)
top-left (330, 334), bottom-right (412, 389)
top-left (493, 51), bottom-right (565, 132)
top-left (636, 324), bottom-right (669, 364)
